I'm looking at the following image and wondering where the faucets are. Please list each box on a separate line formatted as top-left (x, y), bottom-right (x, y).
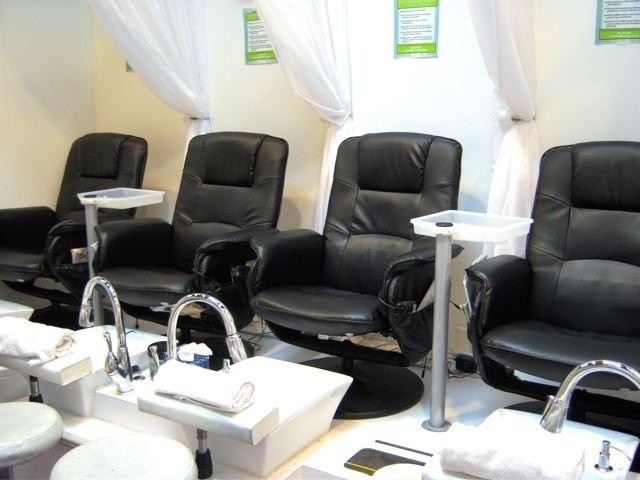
top-left (165, 293), bottom-right (248, 364)
top-left (540, 358), bottom-right (640, 434)
top-left (76, 277), bottom-right (135, 394)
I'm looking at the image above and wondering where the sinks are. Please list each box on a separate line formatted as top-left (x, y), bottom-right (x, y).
top-left (469, 408), bottom-right (638, 479)
top-left (212, 356), bottom-right (353, 476)
top-left (0, 299), bottom-right (35, 321)
top-left (35, 326), bottom-right (167, 418)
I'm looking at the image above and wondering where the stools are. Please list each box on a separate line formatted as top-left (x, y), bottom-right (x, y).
top-left (464, 135), bottom-right (639, 476)
top-left (0, 401), bottom-right (65, 480)
top-left (49, 434), bottom-right (198, 480)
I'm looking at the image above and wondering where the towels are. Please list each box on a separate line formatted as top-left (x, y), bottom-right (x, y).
top-left (153, 357), bottom-right (254, 408)
top-left (436, 418), bottom-right (587, 478)
top-left (0, 314), bottom-right (79, 369)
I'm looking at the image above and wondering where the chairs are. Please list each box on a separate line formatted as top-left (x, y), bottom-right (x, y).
top-left (1, 131), bottom-right (149, 328)
top-left (257, 130), bottom-right (463, 419)
top-left (89, 131), bottom-right (292, 370)
